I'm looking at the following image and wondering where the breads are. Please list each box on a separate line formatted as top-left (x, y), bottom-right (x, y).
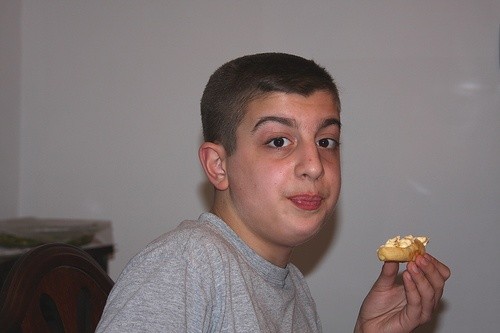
top-left (376, 233), bottom-right (429, 263)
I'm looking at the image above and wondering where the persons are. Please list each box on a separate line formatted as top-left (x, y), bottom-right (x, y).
top-left (88, 50), bottom-right (451, 333)
